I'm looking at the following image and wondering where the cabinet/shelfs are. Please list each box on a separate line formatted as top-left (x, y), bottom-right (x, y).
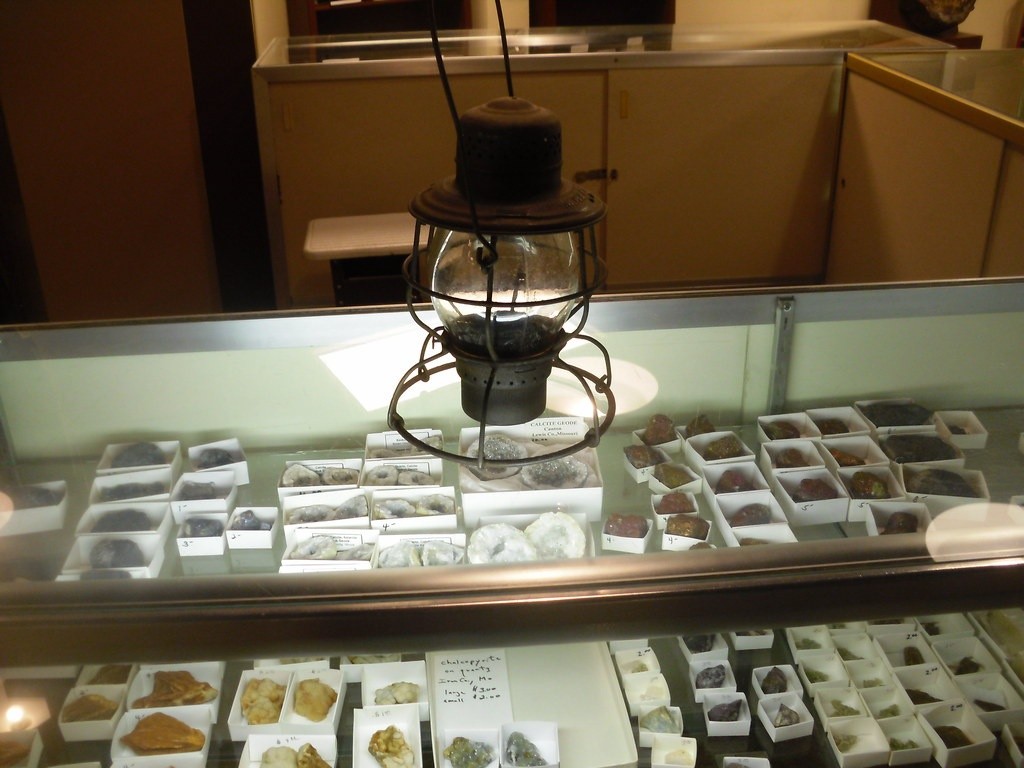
top-left (266, 64), bottom-right (845, 285)
top-left (0, 285), bottom-right (1024, 768)
top-left (823, 71), bottom-right (1023, 284)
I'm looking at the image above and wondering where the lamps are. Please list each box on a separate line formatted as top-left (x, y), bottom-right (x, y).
top-left (389, 0), bottom-right (615, 468)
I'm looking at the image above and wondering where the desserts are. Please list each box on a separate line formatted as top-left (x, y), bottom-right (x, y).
top-left (0, 391), bottom-right (1024, 768)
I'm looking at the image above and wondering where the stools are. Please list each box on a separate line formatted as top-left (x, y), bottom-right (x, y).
top-left (302, 212), bottom-right (437, 306)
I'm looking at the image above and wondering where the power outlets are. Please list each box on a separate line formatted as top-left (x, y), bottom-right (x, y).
top-left (88, 467), bottom-right (174, 501)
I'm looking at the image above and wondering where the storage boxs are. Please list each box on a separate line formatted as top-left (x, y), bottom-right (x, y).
top-left (73, 503), bottom-right (173, 544)
top-left (0, 610), bottom-right (1024, 768)
top-left (95, 439), bottom-right (182, 476)
top-left (171, 472), bottom-right (237, 524)
top-left (56, 534), bottom-right (164, 581)
top-left (0, 479), bottom-right (68, 536)
top-left (187, 437), bottom-right (249, 486)
top-left (225, 506), bottom-right (279, 549)
top-left (176, 512), bottom-right (229, 557)
top-left (276, 397), bottom-right (990, 571)
